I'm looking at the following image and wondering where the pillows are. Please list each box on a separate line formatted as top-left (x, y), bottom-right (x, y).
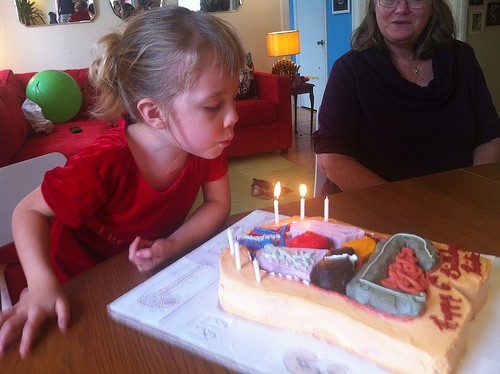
top-left (233, 52), bottom-right (258, 98)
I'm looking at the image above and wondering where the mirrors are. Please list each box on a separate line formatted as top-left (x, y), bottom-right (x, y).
top-left (109, 0), bottom-right (243, 20)
top-left (15, 0), bottom-right (96, 28)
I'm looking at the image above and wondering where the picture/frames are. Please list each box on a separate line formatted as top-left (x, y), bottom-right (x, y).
top-left (468, 0), bottom-right (500, 34)
top-left (332, 0), bottom-right (350, 15)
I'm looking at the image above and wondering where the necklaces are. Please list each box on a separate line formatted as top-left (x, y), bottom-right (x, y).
top-left (396, 62), bottom-right (420, 74)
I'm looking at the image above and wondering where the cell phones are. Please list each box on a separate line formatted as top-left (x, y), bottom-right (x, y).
top-left (70, 127), bottom-right (82, 133)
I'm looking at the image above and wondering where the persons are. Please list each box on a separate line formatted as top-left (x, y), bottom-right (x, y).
top-left (57, 0), bottom-right (73, 23)
top-left (310, 0), bottom-right (500, 197)
top-left (0, 4), bottom-right (247, 358)
top-left (67, 1), bottom-right (90, 22)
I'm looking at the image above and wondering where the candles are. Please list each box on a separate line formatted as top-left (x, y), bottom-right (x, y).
top-left (228, 229), bottom-right (234, 254)
top-left (234, 241), bottom-right (241, 270)
top-left (253, 257), bottom-right (261, 281)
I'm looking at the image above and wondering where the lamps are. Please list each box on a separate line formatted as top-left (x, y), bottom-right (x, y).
top-left (300, 184), bottom-right (307, 220)
top-left (266, 29), bottom-right (301, 85)
top-left (324, 195), bottom-right (329, 223)
top-left (273, 181), bottom-right (281, 225)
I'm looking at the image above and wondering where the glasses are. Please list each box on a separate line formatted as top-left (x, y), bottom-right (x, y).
top-left (379, 0), bottom-right (431, 9)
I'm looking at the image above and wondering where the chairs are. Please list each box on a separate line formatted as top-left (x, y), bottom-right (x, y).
top-left (0, 152), bottom-right (69, 247)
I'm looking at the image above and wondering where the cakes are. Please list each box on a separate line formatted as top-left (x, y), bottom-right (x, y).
top-left (217, 215), bottom-right (493, 374)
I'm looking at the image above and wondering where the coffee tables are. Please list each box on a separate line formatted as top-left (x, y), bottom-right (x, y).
top-left (289, 84), bottom-right (315, 136)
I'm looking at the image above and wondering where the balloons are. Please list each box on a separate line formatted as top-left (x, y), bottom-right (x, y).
top-left (25, 70), bottom-right (82, 124)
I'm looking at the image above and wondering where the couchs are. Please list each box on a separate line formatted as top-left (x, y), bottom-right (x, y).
top-left (0, 68), bottom-right (292, 169)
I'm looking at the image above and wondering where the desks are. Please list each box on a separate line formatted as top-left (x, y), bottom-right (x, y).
top-left (0, 163), bottom-right (500, 374)
